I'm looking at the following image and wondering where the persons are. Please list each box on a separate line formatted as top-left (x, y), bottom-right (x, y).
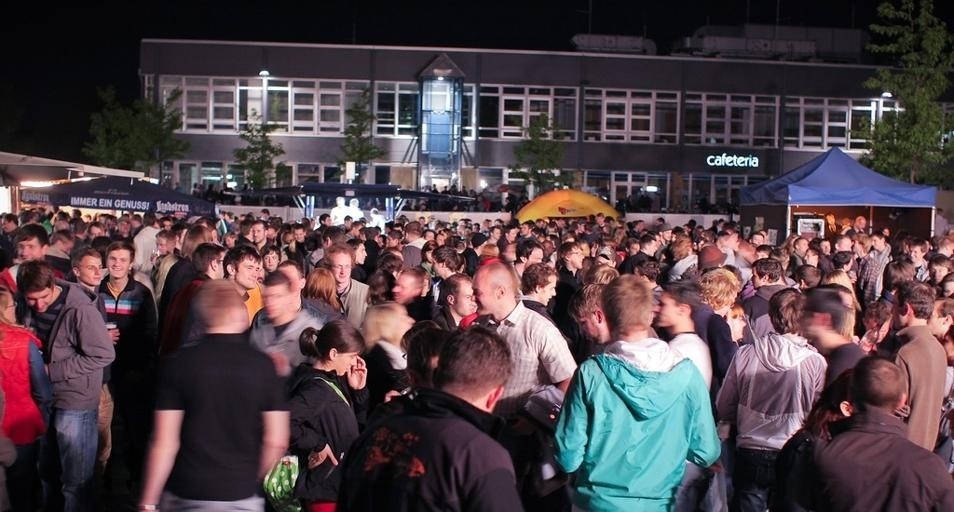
top-left (1, 180), bottom-right (954, 512)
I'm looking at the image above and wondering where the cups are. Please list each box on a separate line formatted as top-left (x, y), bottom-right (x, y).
top-left (152, 249), bottom-right (158, 256)
top-left (104, 321), bottom-right (115, 329)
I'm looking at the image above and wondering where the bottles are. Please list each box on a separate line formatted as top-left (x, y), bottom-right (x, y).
top-left (857, 316), bottom-right (886, 353)
